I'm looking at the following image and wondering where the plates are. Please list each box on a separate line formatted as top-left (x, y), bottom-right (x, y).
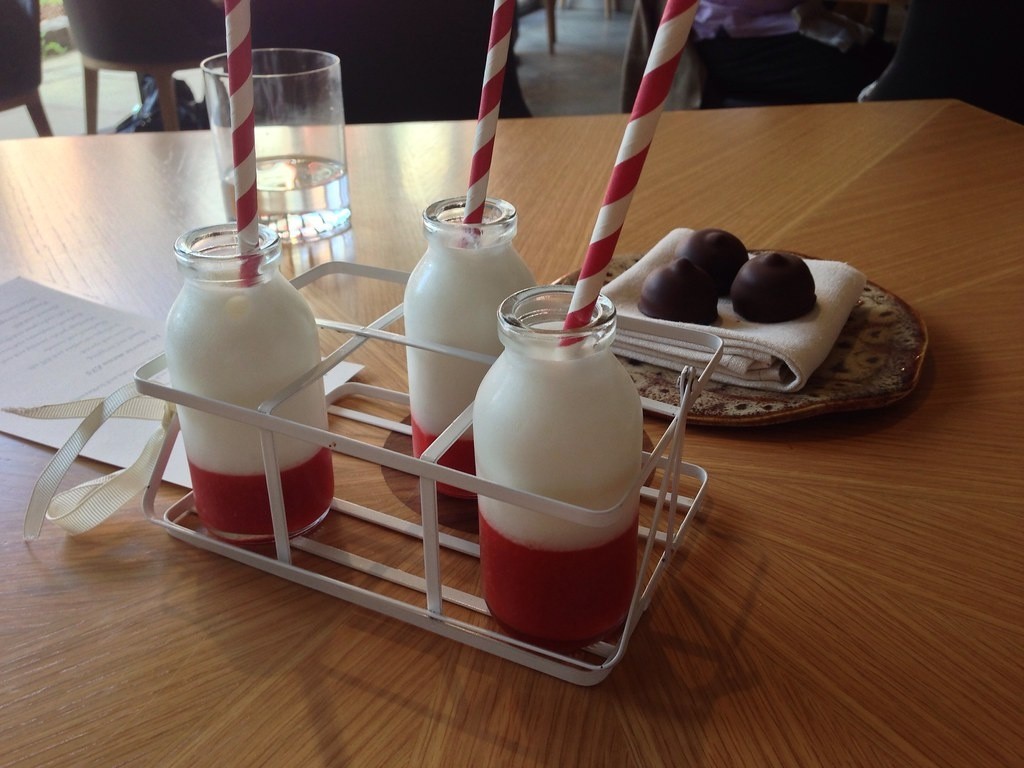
top-left (546, 250), bottom-right (929, 426)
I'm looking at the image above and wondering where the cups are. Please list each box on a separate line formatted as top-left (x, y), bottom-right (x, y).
top-left (201, 47), bottom-right (353, 247)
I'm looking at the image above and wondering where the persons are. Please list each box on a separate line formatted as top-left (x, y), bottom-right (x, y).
top-left (687, 0), bottom-right (885, 112)
top-left (247, 0), bottom-right (536, 123)
top-left (793, 0), bottom-right (1023, 130)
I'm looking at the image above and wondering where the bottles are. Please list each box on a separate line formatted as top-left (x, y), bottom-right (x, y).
top-left (163, 220), bottom-right (334, 541)
top-left (404, 200), bottom-right (536, 498)
top-left (473, 285), bottom-right (642, 642)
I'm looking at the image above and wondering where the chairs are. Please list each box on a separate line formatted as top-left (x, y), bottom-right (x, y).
top-left (0, 0), bottom-right (55, 137)
top-left (62, 0), bottom-right (228, 133)
